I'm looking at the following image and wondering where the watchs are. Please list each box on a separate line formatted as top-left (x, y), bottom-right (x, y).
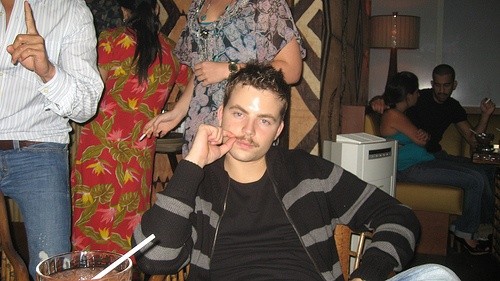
top-left (228, 61), bottom-right (238, 73)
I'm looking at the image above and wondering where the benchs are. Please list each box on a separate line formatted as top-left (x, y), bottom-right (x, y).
top-left (341, 104), bottom-right (500, 256)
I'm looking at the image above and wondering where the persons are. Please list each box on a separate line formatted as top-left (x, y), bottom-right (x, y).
top-left (366, 65), bottom-right (495, 257)
top-left (130, 62), bottom-right (461, 281)
top-left (0, 0), bottom-right (307, 281)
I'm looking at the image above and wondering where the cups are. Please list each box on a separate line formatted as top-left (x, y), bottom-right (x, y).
top-left (476, 133), bottom-right (495, 152)
top-left (36, 251), bottom-right (132, 281)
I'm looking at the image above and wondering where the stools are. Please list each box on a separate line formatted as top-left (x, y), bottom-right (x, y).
top-left (156, 132), bottom-right (187, 174)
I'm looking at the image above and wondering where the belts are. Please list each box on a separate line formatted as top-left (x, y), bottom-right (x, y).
top-left (0, 140), bottom-right (44, 151)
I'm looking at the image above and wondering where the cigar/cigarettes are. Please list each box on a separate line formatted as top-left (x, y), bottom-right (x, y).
top-left (139, 128), bottom-right (150, 141)
top-left (485, 96), bottom-right (491, 104)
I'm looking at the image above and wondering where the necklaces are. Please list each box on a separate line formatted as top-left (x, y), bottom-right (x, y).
top-left (201, 0), bottom-right (212, 20)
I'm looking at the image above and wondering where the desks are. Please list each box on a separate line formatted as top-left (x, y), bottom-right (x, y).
top-left (470, 143), bottom-right (500, 254)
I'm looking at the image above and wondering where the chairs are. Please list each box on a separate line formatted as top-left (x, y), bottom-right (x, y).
top-left (150, 224), bottom-right (395, 281)
top-left (0, 188), bottom-right (30, 281)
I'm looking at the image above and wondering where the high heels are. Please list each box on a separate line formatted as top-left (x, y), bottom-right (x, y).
top-left (450, 230), bottom-right (457, 249)
top-left (456, 234), bottom-right (487, 255)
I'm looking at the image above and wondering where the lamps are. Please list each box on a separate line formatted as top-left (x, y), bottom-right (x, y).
top-left (369, 12), bottom-right (422, 90)
